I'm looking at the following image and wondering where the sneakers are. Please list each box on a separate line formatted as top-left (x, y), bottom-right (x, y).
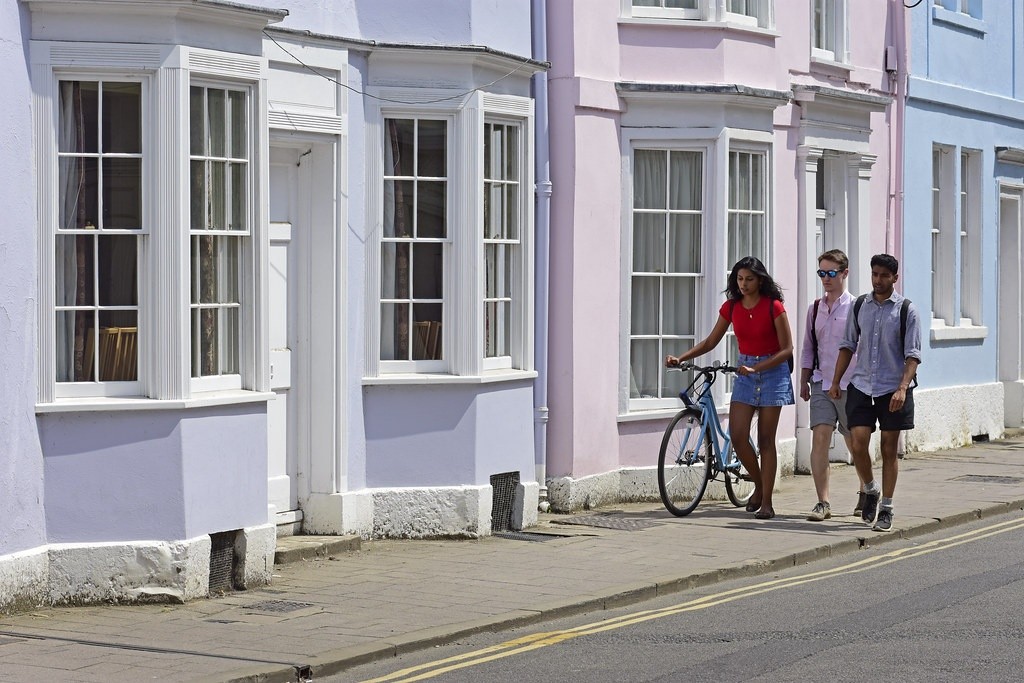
top-left (872, 510), bottom-right (893, 532)
top-left (807, 501), bottom-right (831, 521)
top-left (862, 487), bottom-right (880, 523)
top-left (853, 490), bottom-right (867, 516)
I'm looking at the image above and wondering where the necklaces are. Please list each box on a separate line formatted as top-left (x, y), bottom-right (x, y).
top-left (745, 302), bottom-right (752, 319)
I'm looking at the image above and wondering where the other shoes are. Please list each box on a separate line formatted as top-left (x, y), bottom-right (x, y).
top-left (755, 507), bottom-right (774, 518)
top-left (746, 492), bottom-right (762, 512)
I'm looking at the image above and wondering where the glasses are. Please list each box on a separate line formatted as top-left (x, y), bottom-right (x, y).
top-left (817, 269), bottom-right (843, 277)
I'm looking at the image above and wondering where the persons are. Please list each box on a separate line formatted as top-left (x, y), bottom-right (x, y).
top-left (666, 256), bottom-right (795, 518)
top-left (801, 250), bottom-right (863, 520)
top-left (830, 254), bottom-right (922, 531)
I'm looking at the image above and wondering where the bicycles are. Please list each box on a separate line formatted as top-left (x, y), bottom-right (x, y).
top-left (656, 360), bottom-right (762, 518)
